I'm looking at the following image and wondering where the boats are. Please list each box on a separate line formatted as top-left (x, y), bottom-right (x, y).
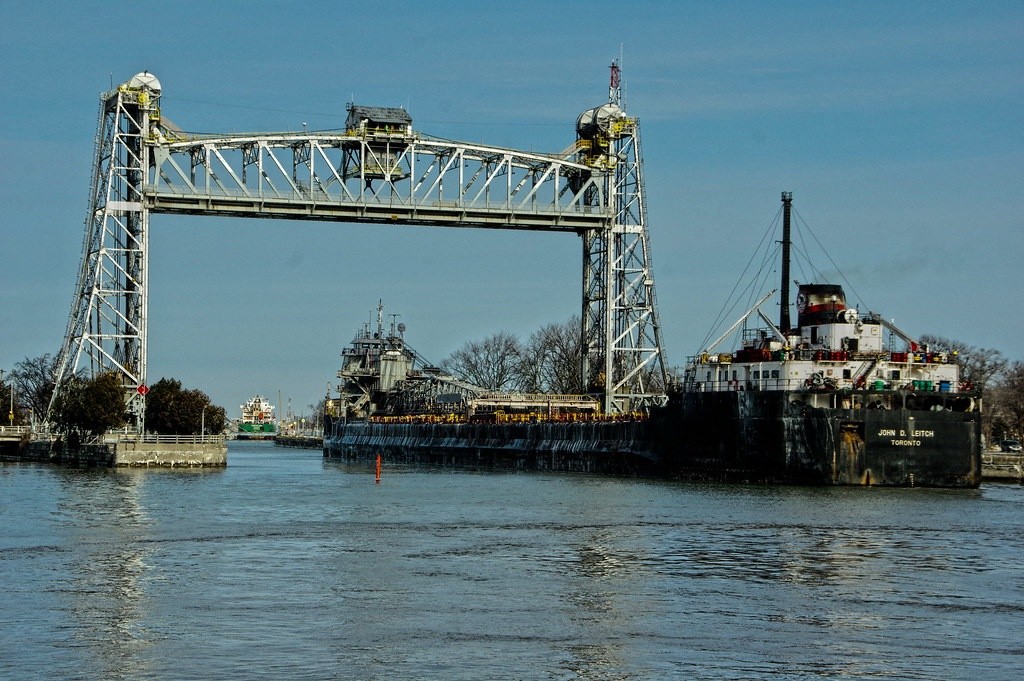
top-left (232, 396), bottom-right (277, 441)
top-left (321, 191), bottom-right (984, 490)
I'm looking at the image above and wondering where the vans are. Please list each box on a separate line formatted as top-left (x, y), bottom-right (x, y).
top-left (1000, 440), bottom-right (1022, 452)
